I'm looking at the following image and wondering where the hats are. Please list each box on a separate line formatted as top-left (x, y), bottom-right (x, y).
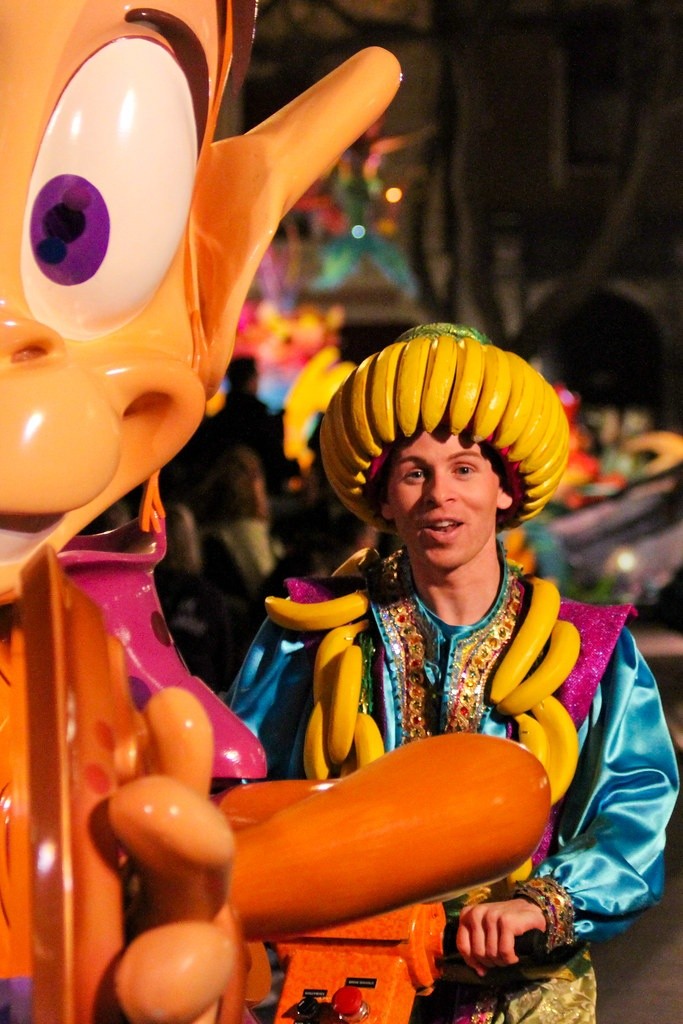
top-left (320, 324), bottom-right (571, 534)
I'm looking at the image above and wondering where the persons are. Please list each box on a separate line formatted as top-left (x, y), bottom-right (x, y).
top-left (220, 323), bottom-right (680, 1024)
top-left (203, 357), bottom-right (274, 590)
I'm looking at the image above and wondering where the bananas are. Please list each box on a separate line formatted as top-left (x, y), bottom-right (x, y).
top-left (263, 549), bottom-right (578, 895)
top-left (318, 334), bottom-right (569, 531)
